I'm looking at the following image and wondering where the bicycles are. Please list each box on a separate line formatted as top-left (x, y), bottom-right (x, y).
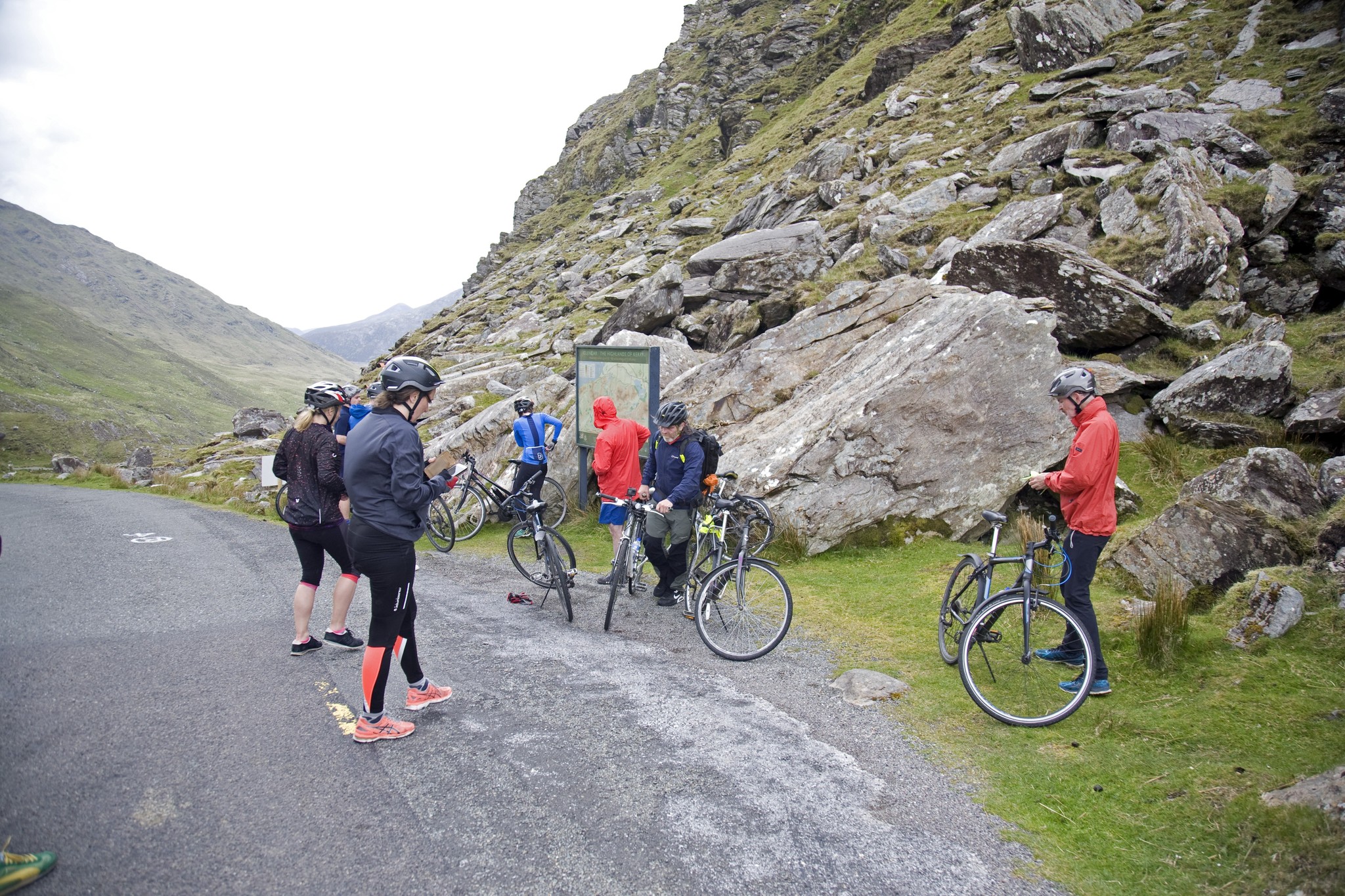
top-left (424, 449), bottom-right (567, 553)
top-left (939, 510), bottom-right (1096, 726)
top-left (690, 470), bottom-right (774, 561)
top-left (683, 492), bottom-right (793, 661)
top-left (500, 470), bottom-right (578, 621)
top-left (596, 487), bottom-right (673, 630)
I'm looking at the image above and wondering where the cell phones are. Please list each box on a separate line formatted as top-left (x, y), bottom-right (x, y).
top-left (447, 463), bottom-right (468, 477)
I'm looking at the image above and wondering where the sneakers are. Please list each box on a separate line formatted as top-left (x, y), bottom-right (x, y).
top-left (0, 835), bottom-right (57, 896)
top-left (653, 576), bottom-right (668, 598)
top-left (657, 588), bottom-right (685, 607)
top-left (1035, 646), bottom-right (1085, 667)
top-left (323, 627), bottom-right (365, 649)
top-left (404, 677), bottom-right (454, 710)
top-left (290, 633), bottom-right (323, 655)
top-left (353, 714), bottom-right (416, 743)
top-left (508, 527), bottom-right (533, 538)
top-left (597, 567), bottom-right (629, 584)
top-left (1058, 671), bottom-right (1112, 696)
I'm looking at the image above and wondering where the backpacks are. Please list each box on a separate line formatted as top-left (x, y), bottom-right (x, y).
top-left (655, 428), bottom-right (724, 492)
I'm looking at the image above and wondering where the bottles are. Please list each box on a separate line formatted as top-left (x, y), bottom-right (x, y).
top-left (632, 537), bottom-right (641, 563)
top-left (490, 486), bottom-right (507, 502)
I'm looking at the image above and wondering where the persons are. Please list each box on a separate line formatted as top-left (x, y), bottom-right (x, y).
top-left (272, 381), bottom-right (364, 655)
top-left (1028, 367), bottom-right (1120, 695)
top-left (333, 382), bottom-right (387, 527)
top-left (592, 396), bottom-right (650, 586)
top-left (342, 356), bottom-right (459, 743)
top-left (638, 401), bottom-right (704, 607)
top-left (511, 396), bottom-right (563, 539)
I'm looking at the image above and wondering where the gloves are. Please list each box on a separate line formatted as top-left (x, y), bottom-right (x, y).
top-left (507, 592), bottom-right (532, 605)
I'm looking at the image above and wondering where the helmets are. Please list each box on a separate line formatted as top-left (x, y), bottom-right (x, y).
top-left (341, 384), bottom-right (362, 398)
top-left (380, 356), bottom-right (446, 392)
top-left (652, 400), bottom-right (688, 428)
top-left (1046, 367), bottom-right (1097, 399)
top-left (304, 381), bottom-right (351, 408)
top-left (514, 396), bottom-right (534, 412)
top-left (366, 382), bottom-right (383, 399)
